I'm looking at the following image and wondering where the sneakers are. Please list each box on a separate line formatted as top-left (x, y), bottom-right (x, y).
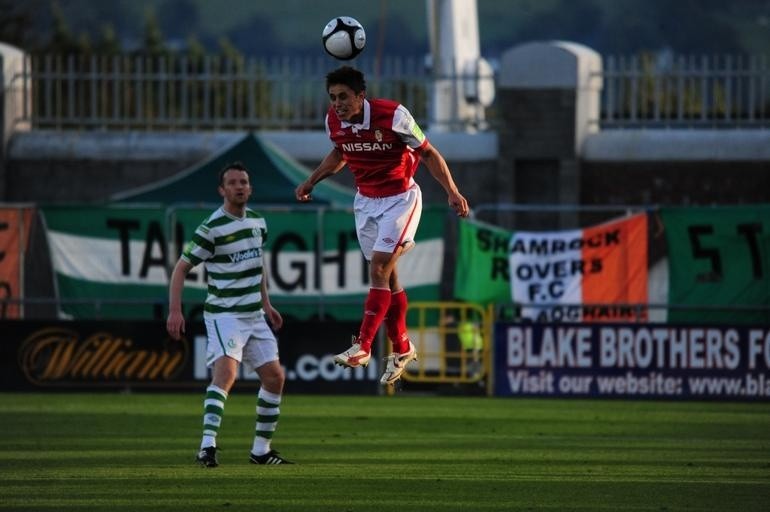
top-left (380, 341), bottom-right (417, 385)
top-left (249, 450), bottom-right (295, 465)
top-left (362, 355), bottom-right (372, 356)
top-left (333, 343), bottom-right (371, 369)
top-left (196, 448), bottom-right (218, 469)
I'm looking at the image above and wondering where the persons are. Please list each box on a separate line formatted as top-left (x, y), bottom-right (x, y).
top-left (166, 162), bottom-right (296, 468)
top-left (294, 67), bottom-right (469, 385)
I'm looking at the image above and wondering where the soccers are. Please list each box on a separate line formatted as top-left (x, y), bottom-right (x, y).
top-left (322, 16), bottom-right (366, 60)
